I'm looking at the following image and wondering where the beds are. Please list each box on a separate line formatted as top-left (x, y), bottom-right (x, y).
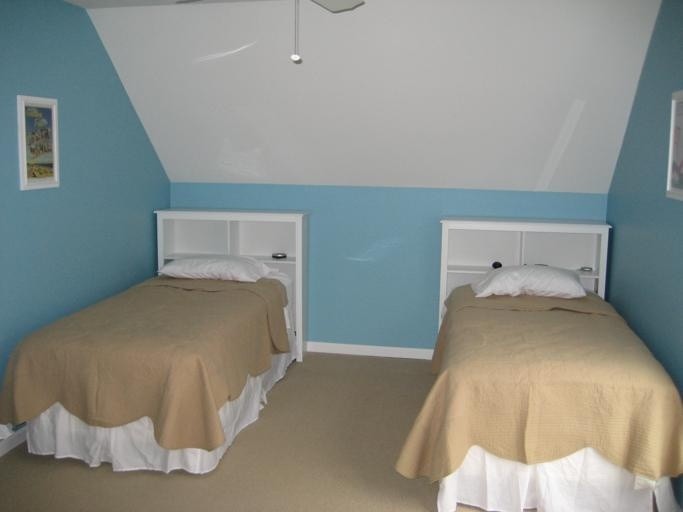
top-left (0, 271), bottom-right (297, 476)
top-left (395, 283), bottom-right (683, 511)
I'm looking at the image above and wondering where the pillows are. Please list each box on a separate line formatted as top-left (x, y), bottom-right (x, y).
top-left (469, 265), bottom-right (589, 300)
top-left (158, 254), bottom-right (270, 284)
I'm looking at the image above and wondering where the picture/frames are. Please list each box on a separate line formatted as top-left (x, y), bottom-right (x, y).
top-left (16, 93), bottom-right (61, 192)
top-left (663, 88), bottom-right (683, 202)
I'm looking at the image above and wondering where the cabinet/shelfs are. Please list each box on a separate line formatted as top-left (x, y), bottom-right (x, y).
top-left (151, 209), bottom-right (305, 362)
top-left (438, 215), bottom-right (614, 336)
